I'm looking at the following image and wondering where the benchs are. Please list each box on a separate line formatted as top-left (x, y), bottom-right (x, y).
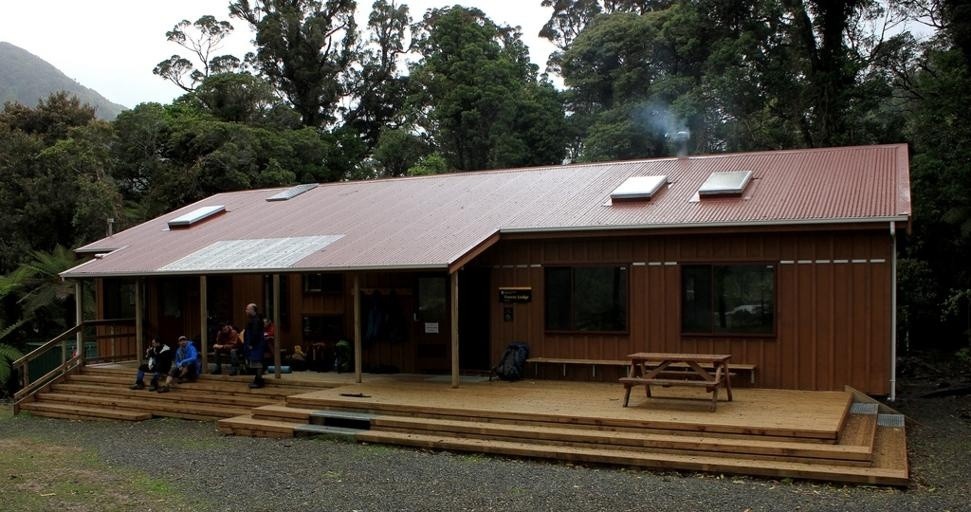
top-left (525, 357), bottom-right (758, 412)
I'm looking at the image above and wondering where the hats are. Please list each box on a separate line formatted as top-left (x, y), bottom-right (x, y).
top-left (178, 336), bottom-right (187, 341)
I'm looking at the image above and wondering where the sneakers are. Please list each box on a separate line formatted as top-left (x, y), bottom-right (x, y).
top-left (210, 364), bottom-right (239, 375)
top-left (128, 377), bottom-right (182, 394)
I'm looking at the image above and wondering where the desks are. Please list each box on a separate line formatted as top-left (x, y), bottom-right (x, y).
top-left (623, 352), bottom-right (734, 412)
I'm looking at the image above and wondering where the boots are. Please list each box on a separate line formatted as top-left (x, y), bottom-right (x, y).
top-left (248, 376), bottom-right (265, 388)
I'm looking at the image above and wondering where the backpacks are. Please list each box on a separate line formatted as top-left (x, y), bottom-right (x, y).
top-left (307, 340), bottom-right (334, 372)
top-left (335, 339), bottom-right (354, 374)
top-left (488, 341), bottom-right (528, 383)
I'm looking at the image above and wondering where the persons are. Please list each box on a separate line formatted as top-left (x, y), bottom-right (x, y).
top-left (238, 318), bottom-right (297, 366)
top-left (157, 336), bottom-right (201, 394)
top-left (209, 323), bottom-right (243, 376)
top-left (128, 335), bottom-right (172, 391)
top-left (242, 303), bottom-right (268, 388)
